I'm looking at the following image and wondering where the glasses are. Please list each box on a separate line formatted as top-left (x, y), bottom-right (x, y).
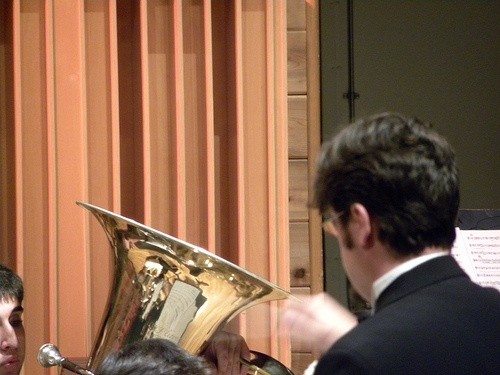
top-left (321, 208), bottom-right (347, 236)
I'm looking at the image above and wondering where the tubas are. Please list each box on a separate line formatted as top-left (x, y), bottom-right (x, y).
top-left (37, 201), bottom-right (294, 374)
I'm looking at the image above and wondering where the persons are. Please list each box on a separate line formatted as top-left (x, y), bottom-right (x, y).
top-left (281, 112), bottom-right (500, 375)
top-left (0, 264), bottom-right (251, 375)
